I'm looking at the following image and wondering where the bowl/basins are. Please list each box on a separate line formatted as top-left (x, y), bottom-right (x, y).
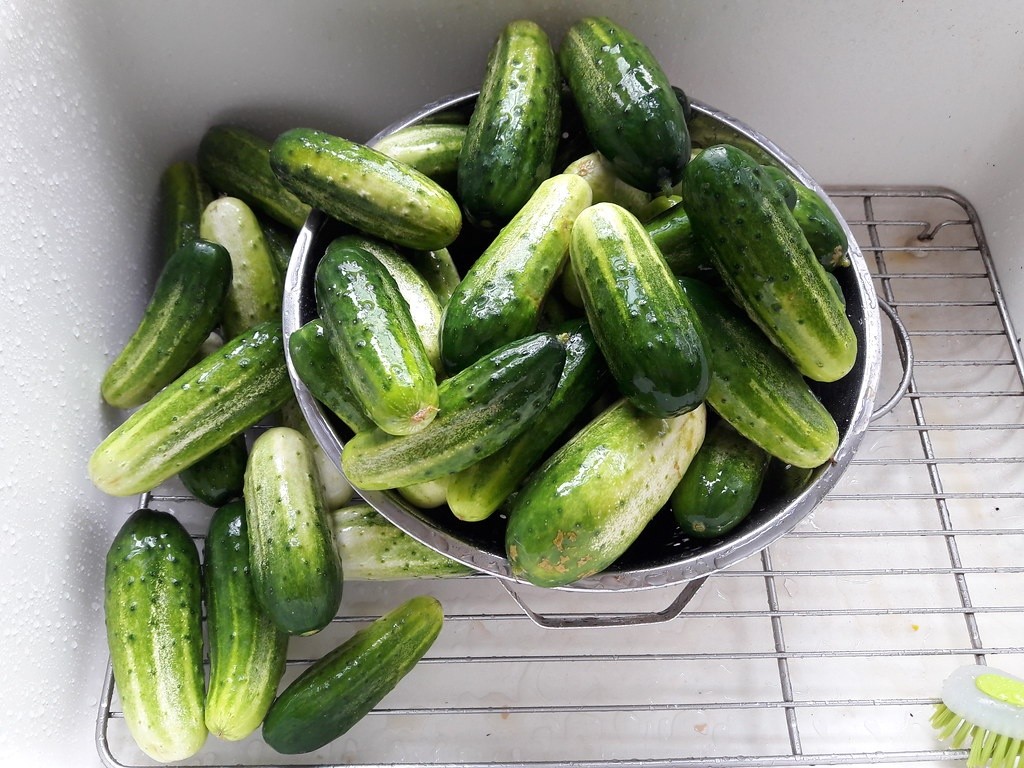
top-left (281, 84), bottom-right (917, 630)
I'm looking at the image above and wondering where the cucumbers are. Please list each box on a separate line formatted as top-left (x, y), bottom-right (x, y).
top-left (90, 15), bottom-right (856, 761)
